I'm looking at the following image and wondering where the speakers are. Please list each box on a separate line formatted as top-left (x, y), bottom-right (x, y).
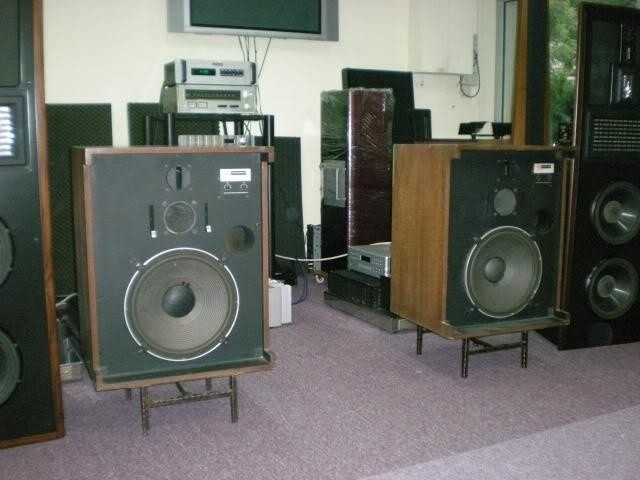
top-left (69, 145), bottom-right (277, 391)
top-left (1, 0), bottom-right (66, 450)
top-left (393, 143), bottom-right (570, 339)
top-left (533, 158), bottom-right (640, 351)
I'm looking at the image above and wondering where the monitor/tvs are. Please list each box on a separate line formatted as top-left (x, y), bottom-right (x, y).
top-left (165, 0), bottom-right (339, 42)
